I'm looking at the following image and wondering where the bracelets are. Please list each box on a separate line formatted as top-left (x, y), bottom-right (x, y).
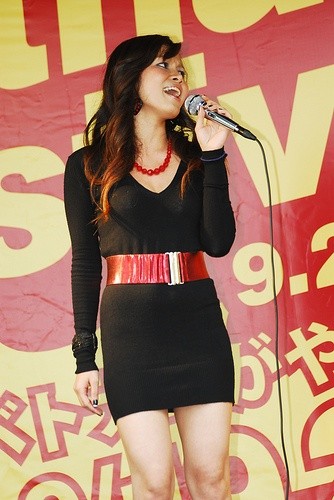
top-left (200, 152), bottom-right (227, 161)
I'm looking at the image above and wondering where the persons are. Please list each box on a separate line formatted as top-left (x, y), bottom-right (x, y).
top-left (63, 34), bottom-right (237, 500)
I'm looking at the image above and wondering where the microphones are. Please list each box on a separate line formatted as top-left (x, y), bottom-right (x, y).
top-left (185, 94), bottom-right (256, 142)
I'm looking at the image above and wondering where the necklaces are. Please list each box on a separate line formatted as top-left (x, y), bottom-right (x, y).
top-left (133, 138), bottom-right (172, 176)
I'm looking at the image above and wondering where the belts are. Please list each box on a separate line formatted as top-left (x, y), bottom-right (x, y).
top-left (104, 250), bottom-right (210, 286)
